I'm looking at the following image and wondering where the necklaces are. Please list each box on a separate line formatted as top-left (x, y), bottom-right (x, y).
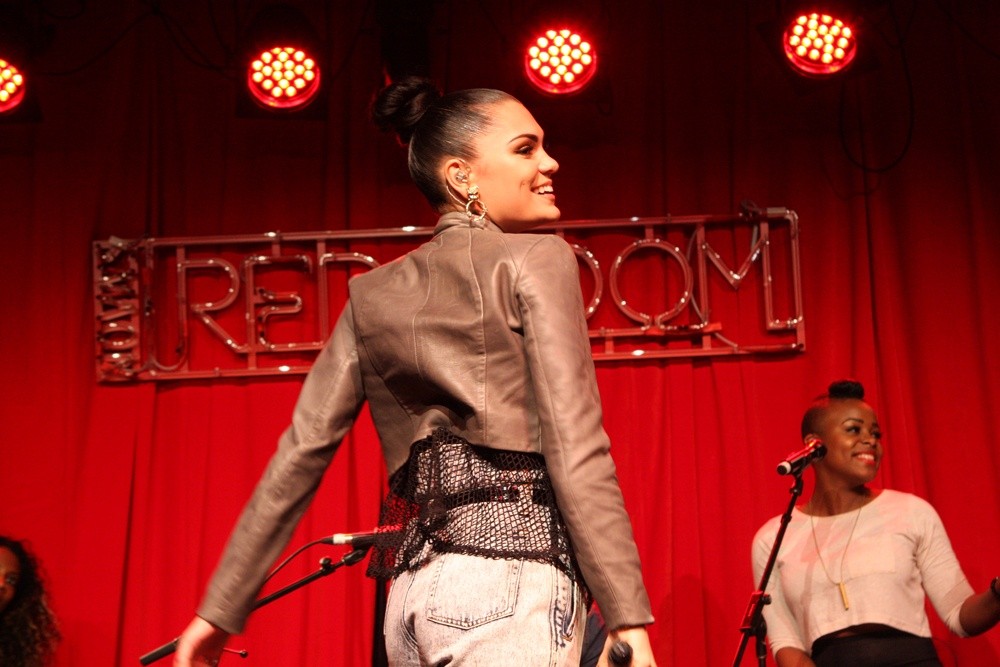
top-left (808, 501), bottom-right (862, 610)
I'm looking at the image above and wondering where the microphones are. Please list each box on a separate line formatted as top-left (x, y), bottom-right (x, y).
top-left (777, 443), bottom-right (829, 475)
top-left (323, 522), bottom-right (403, 546)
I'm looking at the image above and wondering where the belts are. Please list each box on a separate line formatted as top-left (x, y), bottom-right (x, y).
top-left (444, 487), bottom-right (556, 506)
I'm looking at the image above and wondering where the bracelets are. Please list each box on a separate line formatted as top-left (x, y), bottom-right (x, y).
top-left (990, 576), bottom-right (1000, 602)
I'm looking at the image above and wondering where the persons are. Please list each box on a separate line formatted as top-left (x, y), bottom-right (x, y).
top-left (172, 77), bottom-right (656, 667)
top-left (750, 379), bottom-right (1000, 667)
top-left (0, 535), bottom-right (63, 667)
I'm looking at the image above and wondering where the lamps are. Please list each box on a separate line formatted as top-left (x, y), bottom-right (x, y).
top-left (508, 3), bottom-right (606, 102)
top-left (235, 9), bottom-right (329, 119)
top-left (0, 48), bottom-right (40, 123)
top-left (759, 0), bottom-right (879, 95)
